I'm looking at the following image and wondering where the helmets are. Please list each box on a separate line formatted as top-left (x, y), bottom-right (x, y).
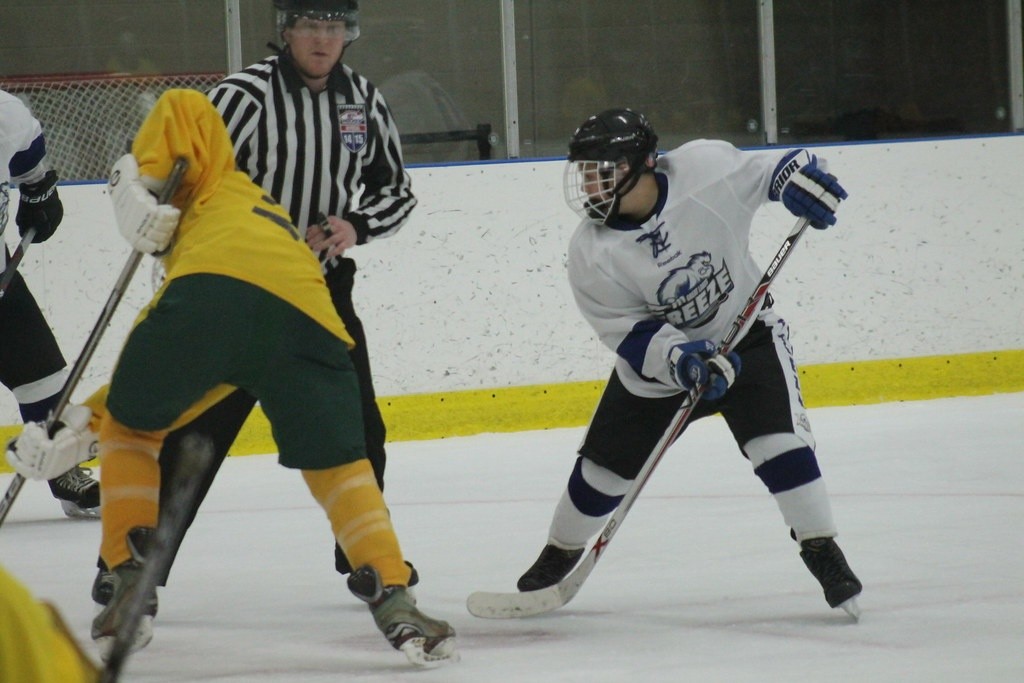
top-left (567, 108), bottom-right (660, 164)
top-left (271, 2), bottom-right (363, 22)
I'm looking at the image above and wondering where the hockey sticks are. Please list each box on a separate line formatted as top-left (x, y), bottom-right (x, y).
top-left (102, 427), bottom-right (215, 682)
top-left (0, 152), bottom-right (189, 521)
top-left (468, 172), bottom-right (839, 619)
top-left (0, 227), bottom-right (36, 298)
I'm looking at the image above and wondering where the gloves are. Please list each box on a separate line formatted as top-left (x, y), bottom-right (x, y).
top-left (664, 338), bottom-right (742, 401)
top-left (767, 147), bottom-right (848, 231)
top-left (102, 154), bottom-right (181, 257)
top-left (3, 406), bottom-right (101, 482)
top-left (15, 170), bottom-right (63, 243)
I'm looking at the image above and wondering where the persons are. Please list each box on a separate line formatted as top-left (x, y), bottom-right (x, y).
top-left (517, 107), bottom-right (862, 623)
top-left (0, 0), bottom-right (458, 683)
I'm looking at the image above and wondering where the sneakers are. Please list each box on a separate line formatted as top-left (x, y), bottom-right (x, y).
top-left (798, 536), bottom-right (864, 625)
top-left (333, 538), bottom-right (420, 589)
top-left (90, 561), bottom-right (158, 665)
top-left (517, 541), bottom-right (584, 592)
top-left (363, 584), bottom-right (457, 671)
top-left (49, 461), bottom-right (102, 520)
